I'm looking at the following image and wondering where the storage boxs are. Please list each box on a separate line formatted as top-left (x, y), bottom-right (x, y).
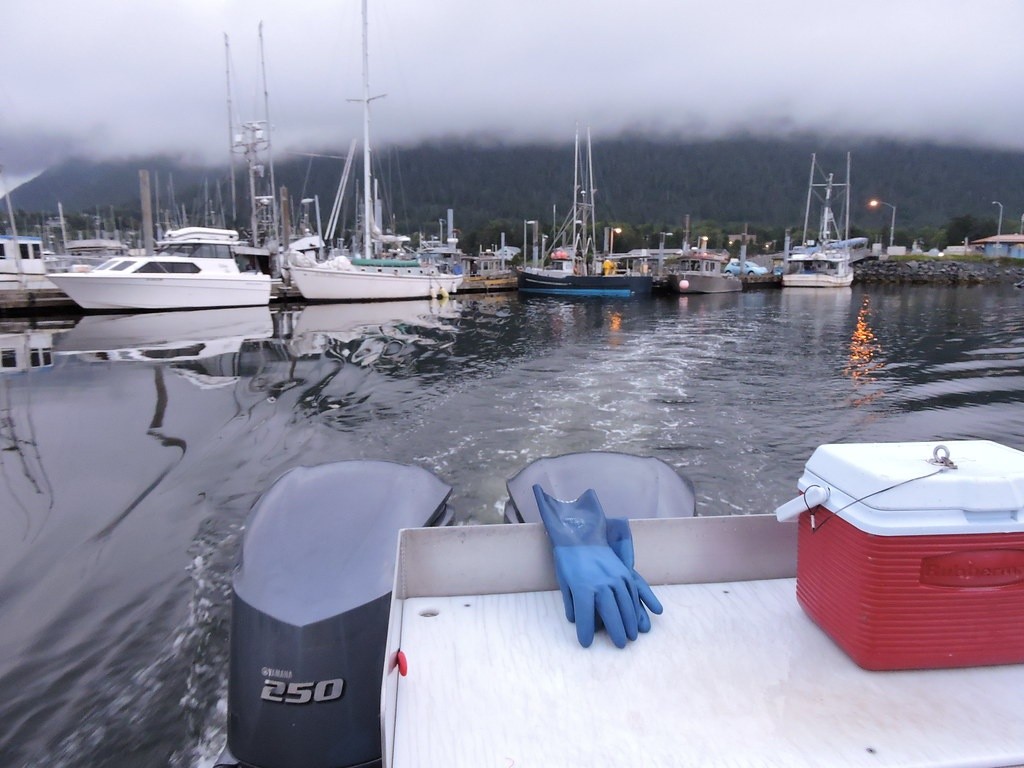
top-left (777, 440), bottom-right (1024, 670)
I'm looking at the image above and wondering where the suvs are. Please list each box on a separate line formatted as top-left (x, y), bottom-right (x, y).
top-left (725, 261), bottom-right (768, 276)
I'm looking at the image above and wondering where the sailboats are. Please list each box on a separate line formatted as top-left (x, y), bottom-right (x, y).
top-left (514, 121), bottom-right (652, 296)
top-left (284, 0), bottom-right (464, 301)
top-left (780, 151), bottom-right (855, 287)
top-left (291, 298), bottom-right (463, 366)
top-left (53, 202), bottom-right (164, 271)
top-left (0, 166), bottom-right (73, 308)
top-left (163, 24), bottom-right (519, 271)
top-left (0, 312), bottom-right (76, 538)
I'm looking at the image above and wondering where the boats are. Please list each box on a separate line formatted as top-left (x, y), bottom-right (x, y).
top-left (666, 253), bottom-right (744, 293)
top-left (46, 225), bottom-right (273, 311)
top-left (52, 307), bottom-right (275, 365)
top-left (457, 256), bottom-right (519, 292)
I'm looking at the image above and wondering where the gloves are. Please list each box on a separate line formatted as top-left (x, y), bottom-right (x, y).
top-left (533, 482), bottom-right (666, 649)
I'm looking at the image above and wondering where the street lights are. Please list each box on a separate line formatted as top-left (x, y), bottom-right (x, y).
top-left (992, 201), bottom-right (1004, 257)
top-left (610, 226), bottom-right (621, 260)
top-left (871, 201), bottom-right (896, 245)
top-left (697, 236), bottom-right (708, 255)
